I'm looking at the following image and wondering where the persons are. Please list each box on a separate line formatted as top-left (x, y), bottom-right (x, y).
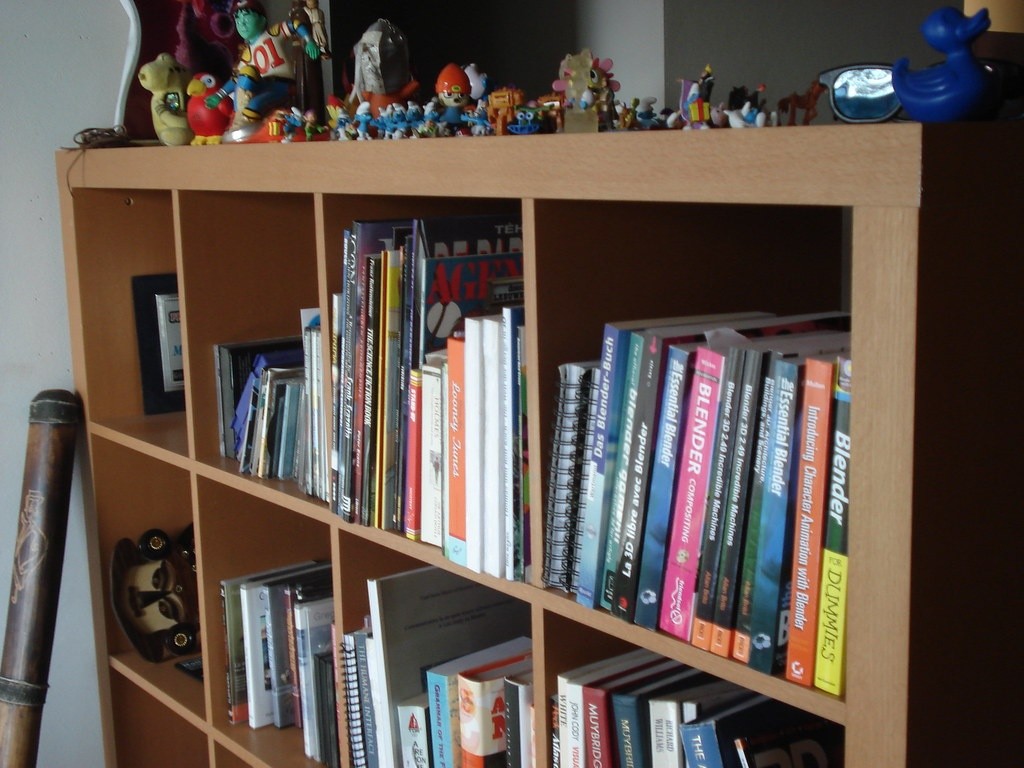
top-left (204, 1), bottom-right (319, 140)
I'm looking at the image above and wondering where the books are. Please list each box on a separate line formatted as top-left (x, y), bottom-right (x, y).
top-left (211, 220), bottom-right (850, 768)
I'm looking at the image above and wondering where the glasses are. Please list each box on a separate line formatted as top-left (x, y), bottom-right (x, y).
top-left (819, 58), bottom-right (1008, 123)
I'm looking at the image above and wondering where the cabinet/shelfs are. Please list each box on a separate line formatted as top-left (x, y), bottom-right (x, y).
top-left (55, 121), bottom-right (1024, 768)
